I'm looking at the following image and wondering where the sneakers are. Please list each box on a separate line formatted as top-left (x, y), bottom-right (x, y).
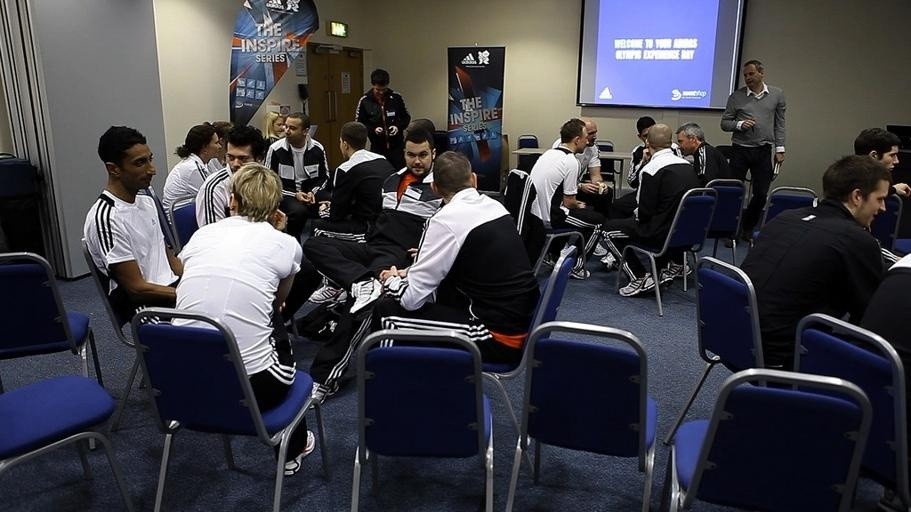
top-left (725, 236), bottom-right (739, 248)
top-left (592, 241), bottom-right (608, 257)
top-left (599, 251), bottom-right (617, 273)
top-left (308, 284), bottom-right (349, 309)
top-left (618, 271), bottom-right (657, 296)
top-left (283, 429), bottom-right (318, 475)
top-left (569, 267), bottom-right (592, 280)
top-left (659, 270), bottom-right (674, 287)
top-left (668, 260), bottom-right (693, 278)
top-left (348, 276), bottom-right (383, 316)
top-left (741, 229), bottom-right (756, 243)
top-left (308, 377), bottom-right (341, 411)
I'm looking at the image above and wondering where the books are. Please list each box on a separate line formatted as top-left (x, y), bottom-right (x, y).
top-left (771, 162), bottom-right (780, 181)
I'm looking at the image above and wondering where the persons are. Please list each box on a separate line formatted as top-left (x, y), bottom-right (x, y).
top-left (854, 128), bottom-right (911, 268)
top-left (84, 123), bottom-right (187, 313)
top-left (161, 109), bottom-right (456, 344)
top-left (739, 155), bottom-right (893, 388)
top-left (354, 67), bottom-right (415, 162)
top-left (520, 114), bottom-right (732, 299)
top-left (306, 150), bottom-right (544, 411)
top-left (172, 162), bottom-right (318, 479)
top-left (862, 253), bottom-right (911, 512)
top-left (720, 59), bottom-right (786, 248)
